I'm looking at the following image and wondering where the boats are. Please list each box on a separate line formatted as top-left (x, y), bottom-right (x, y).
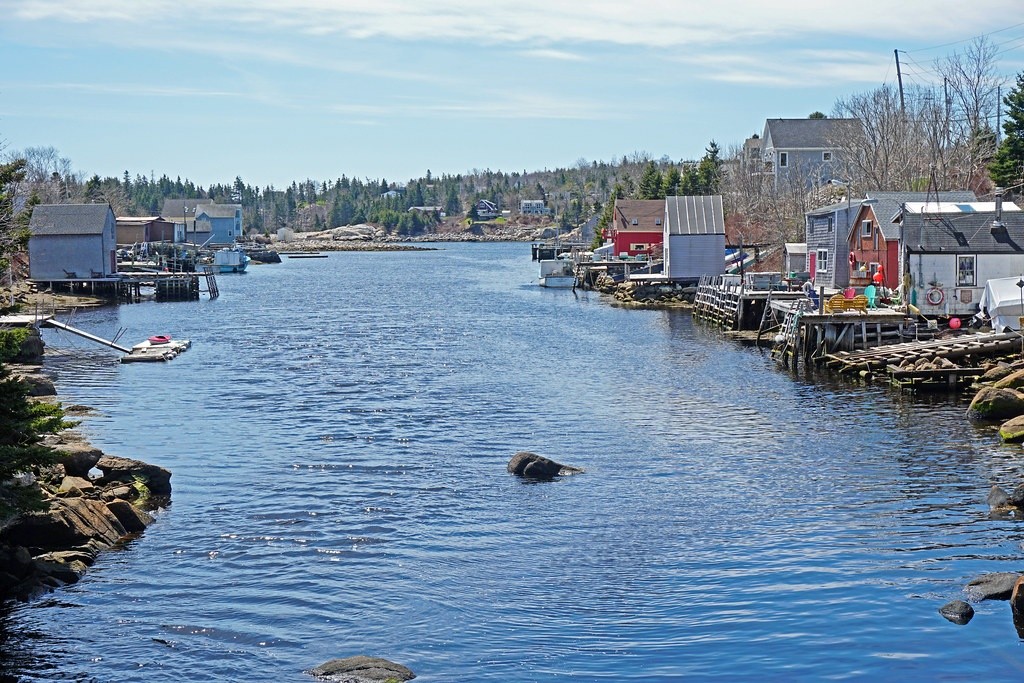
top-left (538, 198), bottom-right (580, 288)
top-left (194, 246), bottom-right (251, 273)
top-left (148, 335), bottom-right (173, 344)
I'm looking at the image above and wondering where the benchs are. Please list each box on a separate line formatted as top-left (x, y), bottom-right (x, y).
top-left (823, 294), bottom-right (868, 314)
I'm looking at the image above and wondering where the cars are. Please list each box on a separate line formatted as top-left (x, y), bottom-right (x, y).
top-left (116, 249), bottom-right (128, 258)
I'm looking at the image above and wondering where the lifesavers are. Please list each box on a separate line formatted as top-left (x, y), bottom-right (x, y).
top-left (802, 281), bottom-right (815, 297)
top-left (925, 287), bottom-right (945, 306)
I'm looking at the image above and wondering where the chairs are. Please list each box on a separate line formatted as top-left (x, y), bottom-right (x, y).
top-left (843, 287), bottom-right (856, 298)
top-left (864, 285), bottom-right (877, 311)
top-left (805, 286), bottom-right (819, 310)
